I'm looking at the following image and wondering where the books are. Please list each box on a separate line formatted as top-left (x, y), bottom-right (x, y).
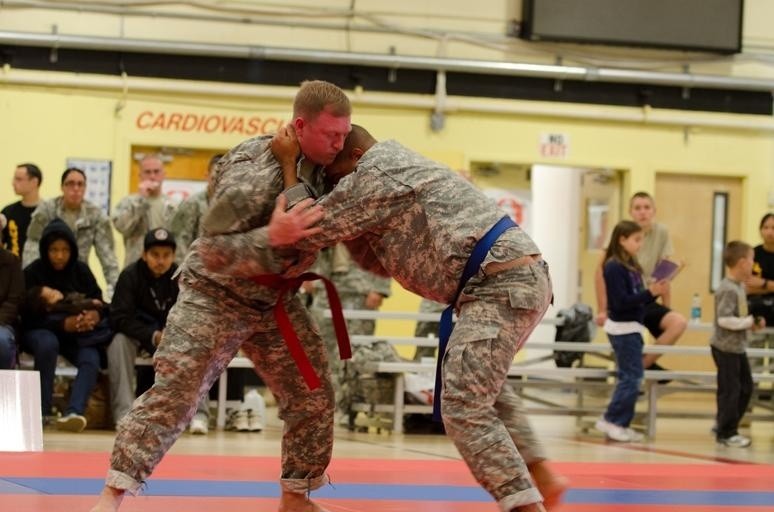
top-left (653, 253), bottom-right (684, 287)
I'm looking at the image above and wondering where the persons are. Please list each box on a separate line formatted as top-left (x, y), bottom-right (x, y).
top-left (300, 242), bottom-right (390, 430)
top-left (88, 79), bottom-right (353, 512)
top-left (596, 191), bottom-right (675, 387)
top-left (17, 218), bottom-right (106, 433)
top-left (111, 157), bottom-right (177, 272)
top-left (0, 165), bottom-right (43, 269)
top-left (410, 297), bottom-right (460, 367)
top-left (175, 155), bottom-right (224, 264)
top-left (745, 212), bottom-right (774, 405)
top-left (25, 169), bottom-right (120, 300)
top-left (711, 239), bottom-right (766, 448)
top-left (0, 212), bottom-right (22, 370)
top-left (104, 227), bottom-right (210, 436)
top-left (271, 124), bottom-right (571, 512)
top-left (595, 220), bottom-right (670, 443)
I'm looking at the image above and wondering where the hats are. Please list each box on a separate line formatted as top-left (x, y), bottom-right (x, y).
top-left (144, 227), bottom-right (177, 252)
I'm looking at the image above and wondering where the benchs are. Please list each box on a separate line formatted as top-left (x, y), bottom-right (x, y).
top-left (19, 358), bottom-right (254, 430)
top-left (539, 314), bottom-right (774, 336)
top-left (522, 341), bottom-right (773, 359)
top-left (374, 361), bottom-right (607, 448)
top-left (347, 335), bottom-right (438, 347)
top-left (642, 369), bottom-right (774, 438)
top-left (319, 308), bottom-right (457, 323)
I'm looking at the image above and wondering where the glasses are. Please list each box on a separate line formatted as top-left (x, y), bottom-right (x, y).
top-left (63, 180), bottom-right (85, 187)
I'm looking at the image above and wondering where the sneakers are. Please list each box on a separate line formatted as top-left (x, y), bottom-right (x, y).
top-left (225, 408), bottom-right (248, 431)
top-left (595, 417), bottom-right (634, 442)
top-left (626, 428), bottom-right (644, 442)
top-left (247, 406), bottom-right (263, 431)
top-left (56, 414), bottom-right (87, 433)
top-left (712, 424), bottom-right (719, 433)
top-left (190, 413), bottom-right (208, 434)
top-left (717, 435), bottom-right (751, 447)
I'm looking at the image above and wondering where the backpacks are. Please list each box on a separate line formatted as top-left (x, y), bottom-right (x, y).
top-left (553, 303), bottom-right (593, 368)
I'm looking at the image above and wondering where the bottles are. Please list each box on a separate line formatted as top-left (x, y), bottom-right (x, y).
top-left (690, 292), bottom-right (701, 326)
